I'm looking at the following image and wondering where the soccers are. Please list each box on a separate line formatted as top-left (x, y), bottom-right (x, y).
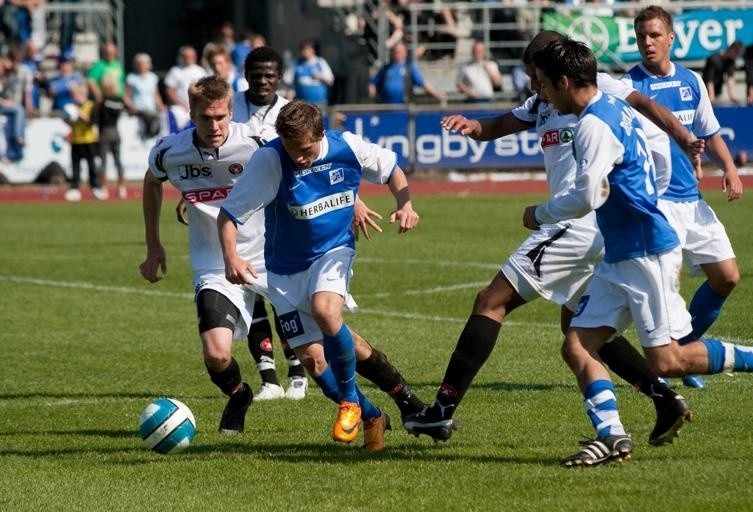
top-left (139, 397), bottom-right (196, 455)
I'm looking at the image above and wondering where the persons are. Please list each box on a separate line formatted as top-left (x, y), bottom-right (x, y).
top-left (615, 5), bottom-right (740, 390)
top-left (176, 47), bottom-right (308, 400)
top-left (347, 0), bottom-right (532, 105)
top-left (401, 31), bottom-right (707, 447)
top-left (137, 76), bottom-right (464, 435)
top-left (1, 0), bottom-right (334, 202)
top-left (521, 38), bottom-right (752, 466)
top-left (218, 100), bottom-right (419, 451)
top-left (701, 41), bottom-right (751, 107)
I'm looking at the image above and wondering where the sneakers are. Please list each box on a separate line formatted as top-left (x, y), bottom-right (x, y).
top-left (219, 382), bottom-right (253, 437)
top-left (401, 395), bottom-right (460, 443)
top-left (253, 381), bottom-right (286, 402)
top-left (681, 374), bottom-right (705, 388)
top-left (648, 394), bottom-right (692, 447)
top-left (332, 399), bottom-right (362, 443)
top-left (286, 376), bottom-right (309, 400)
top-left (363, 406), bottom-right (390, 453)
top-left (560, 435), bottom-right (634, 468)
